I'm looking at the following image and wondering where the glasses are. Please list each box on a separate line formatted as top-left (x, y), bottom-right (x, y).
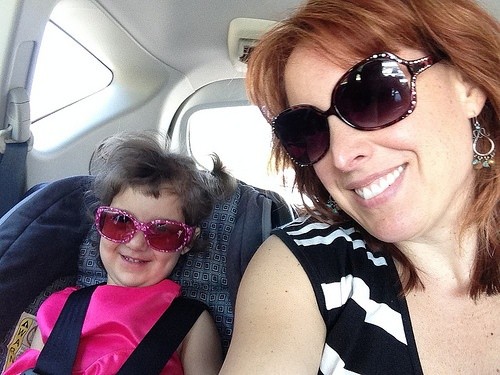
top-left (90, 207), bottom-right (197, 253)
top-left (270, 53), bottom-right (437, 171)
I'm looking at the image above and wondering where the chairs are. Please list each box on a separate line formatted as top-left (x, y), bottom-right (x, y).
top-left (0, 175), bottom-right (299, 375)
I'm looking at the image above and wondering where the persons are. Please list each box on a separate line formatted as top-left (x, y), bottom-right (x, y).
top-left (2, 132), bottom-right (234, 375)
top-left (218, 0), bottom-right (500, 375)
